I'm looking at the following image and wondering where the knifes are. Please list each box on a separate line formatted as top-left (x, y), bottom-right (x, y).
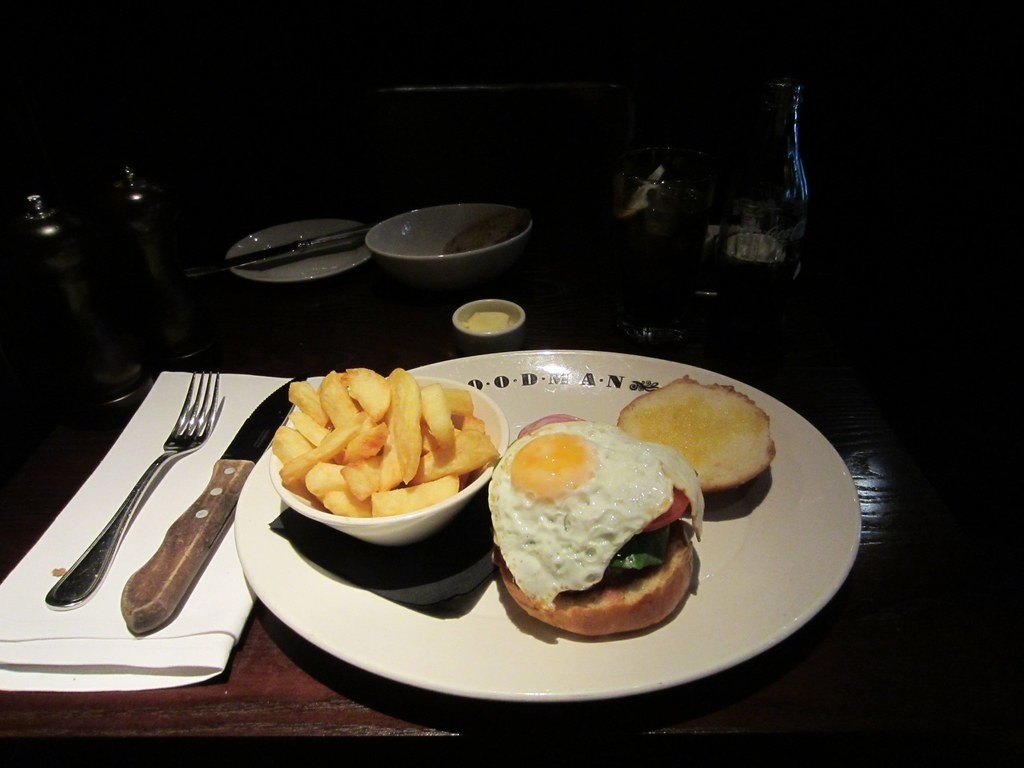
top-left (125, 373), bottom-right (308, 636)
top-left (182, 225), bottom-right (371, 278)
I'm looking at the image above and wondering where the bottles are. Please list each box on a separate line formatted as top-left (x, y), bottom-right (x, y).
top-left (719, 79), bottom-right (811, 334)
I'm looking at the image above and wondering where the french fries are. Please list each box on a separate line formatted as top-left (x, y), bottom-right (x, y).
top-left (272, 367), bottom-right (500, 520)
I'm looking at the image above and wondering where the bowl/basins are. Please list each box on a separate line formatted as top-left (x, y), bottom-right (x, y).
top-left (270, 376), bottom-right (510, 547)
top-left (365, 204), bottom-right (533, 290)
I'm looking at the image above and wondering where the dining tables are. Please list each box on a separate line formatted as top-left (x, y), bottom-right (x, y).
top-left (3, 295), bottom-right (1019, 768)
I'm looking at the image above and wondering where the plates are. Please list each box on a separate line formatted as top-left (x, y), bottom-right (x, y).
top-left (225, 218), bottom-right (373, 282)
top-left (232, 349), bottom-right (860, 705)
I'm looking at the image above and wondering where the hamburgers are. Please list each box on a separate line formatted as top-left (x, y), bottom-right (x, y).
top-left (489, 419), bottom-right (706, 639)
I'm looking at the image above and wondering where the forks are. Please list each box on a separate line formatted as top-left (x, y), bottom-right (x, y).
top-left (46, 370), bottom-right (218, 607)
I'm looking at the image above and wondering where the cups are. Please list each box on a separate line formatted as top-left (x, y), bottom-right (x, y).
top-left (606, 147), bottom-right (715, 345)
top-left (114, 178), bottom-right (151, 223)
top-left (450, 299), bottom-right (527, 356)
top-left (20, 195), bottom-right (65, 239)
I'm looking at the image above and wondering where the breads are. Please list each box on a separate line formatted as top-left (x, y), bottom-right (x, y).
top-left (615, 376), bottom-right (776, 495)
